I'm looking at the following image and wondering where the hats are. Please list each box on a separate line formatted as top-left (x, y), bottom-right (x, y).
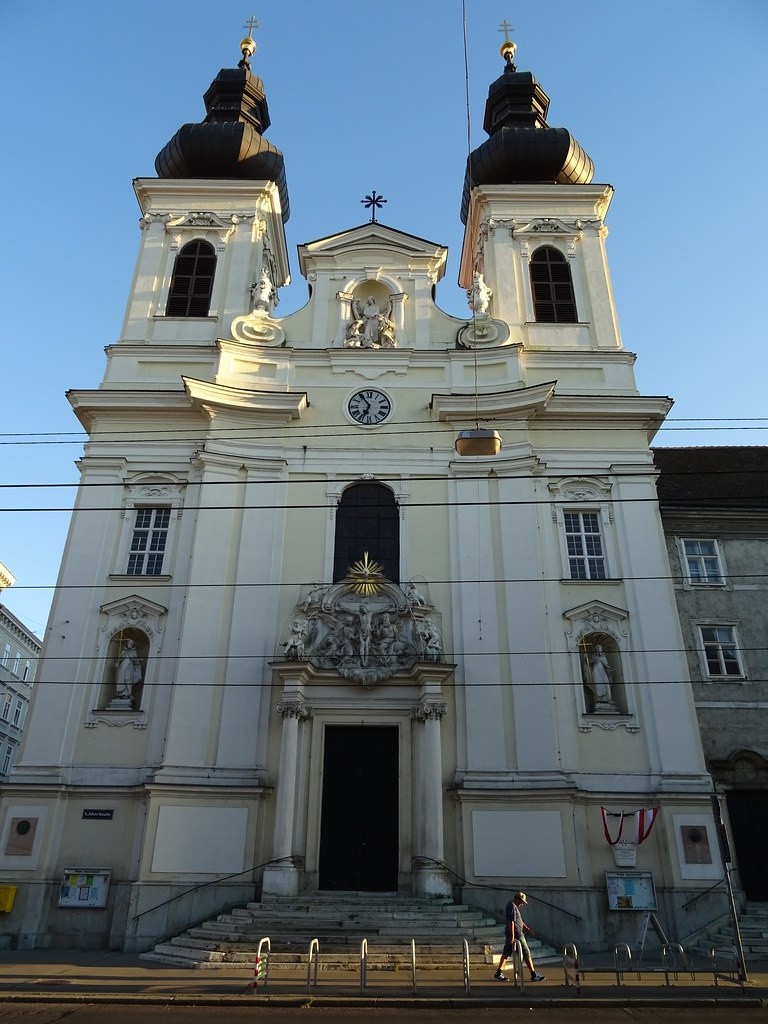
top-left (516, 892), bottom-right (529, 904)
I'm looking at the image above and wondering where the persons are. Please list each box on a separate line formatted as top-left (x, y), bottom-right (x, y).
top-left (585, 645), bottom-right (613, 705)
top-left (114, 640), bottom-right (141, 699)
top-left (282, 580), bottom-right (445, 668)
top-left (492, 892), bottom-right (545, 982)
top-left (362, 296), bottom-right (379, 344)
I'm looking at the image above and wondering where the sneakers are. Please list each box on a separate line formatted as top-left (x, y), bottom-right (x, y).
top-left (494, 972), bottom-right (509, 982)
top-left (531, 976), bottom-right (546, 982)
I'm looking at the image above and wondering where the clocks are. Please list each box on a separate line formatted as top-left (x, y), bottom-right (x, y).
top-left (342, 385), bottom-right (396, 429)
